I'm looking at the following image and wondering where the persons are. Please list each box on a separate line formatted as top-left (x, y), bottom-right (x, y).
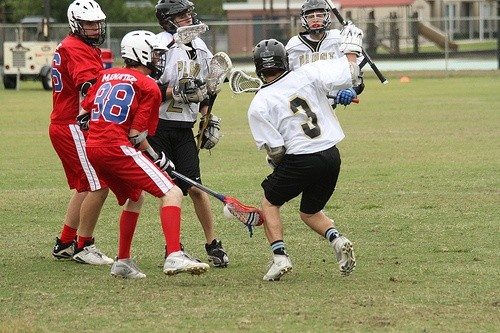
top-left (246, 21), bottom-right (364, 281)
top-left (49, 0), bottom-right (230, 279)
top-left (284, 0), bottom-right (366, 110)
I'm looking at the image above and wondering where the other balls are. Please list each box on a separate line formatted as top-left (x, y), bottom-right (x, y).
top-left (223, 204), bottom-right (236, 219)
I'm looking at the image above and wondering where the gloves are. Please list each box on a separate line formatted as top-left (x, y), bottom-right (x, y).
top-left (334, 87), bottom-right (357, 106)
top-left (198, 114), bottom-right (221, 149)
top-left (151, 150), bottom-right (178, 180)
top-left (339, 20), bottom-right (363, 56)
top-left (171, 77), bottom-right (207, 103)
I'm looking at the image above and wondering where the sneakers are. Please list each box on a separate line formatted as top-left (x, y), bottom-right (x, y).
top-left (53, 235), bottom-right (78, 260)
top-left (72, 237), bottom-right (114, 265)
top-left (111, 256), bottom-right (146, 279)
top-left (205, 239), bottom-right (229, 267)
top-left (331, 234), bottom-right (356, 276)
top-left (163, 253), bottom-right (209, 276)
top-left (164, 243), bottom-right (202, 262)
top-left (263, 259), bottom-right (292, 281)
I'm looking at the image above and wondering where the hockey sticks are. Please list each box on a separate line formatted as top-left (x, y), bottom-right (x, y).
top-left (229, 70), bottom-right (359, 103)
top-left (324, 0), bottom-right (386, 83)
top-left (159, 23), bottom-right (208, 57)
top-left (170, 170), bottom-right (264, 226)
top-left (196, 51), bottom-right (232, 154)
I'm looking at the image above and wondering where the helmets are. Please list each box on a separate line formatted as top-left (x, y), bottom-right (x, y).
top-left (67, 0), bottom-right (106, 46)
top-left (120, 30), bottom-right (169, 79)
top-left (155, 0), bottom-right (198, 34)
top-left (299, 0), bottom-right (331, 34)
top-left (253, 39), bottom-right (290, 77)
